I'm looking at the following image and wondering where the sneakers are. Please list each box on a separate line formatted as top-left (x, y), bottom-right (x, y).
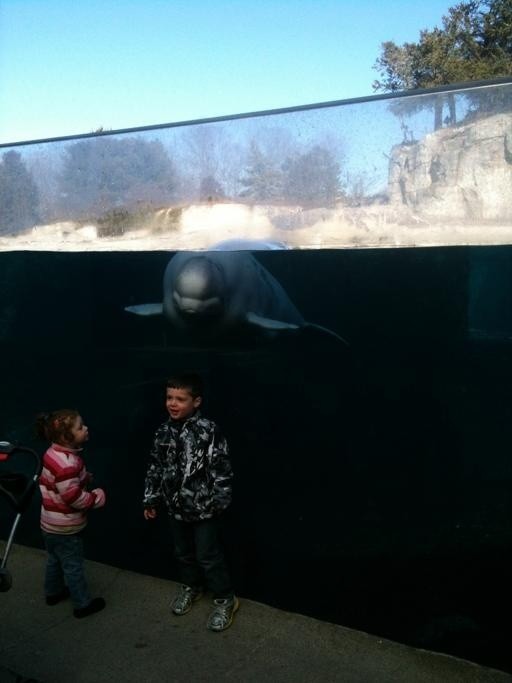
top-left (208, 595), bottom-right (239, 632)
top-left (73, 596), bottom-right (106, 619)
top-left (46, 587), bottom-right (76, 605)
top-left (170, 584), bottom-right (202, 615)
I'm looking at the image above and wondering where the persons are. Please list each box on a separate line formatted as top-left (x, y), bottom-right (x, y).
top-left (36, 405), bottom-right (113, 621)
top-left (142, 369), bottom-right (242, 632)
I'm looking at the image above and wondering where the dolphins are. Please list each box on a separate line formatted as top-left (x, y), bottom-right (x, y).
top-left (123, 239), bottom-right (350, 349)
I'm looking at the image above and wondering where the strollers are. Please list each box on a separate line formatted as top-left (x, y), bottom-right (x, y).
top-left (0, 440), bottom-right (42, 593)
